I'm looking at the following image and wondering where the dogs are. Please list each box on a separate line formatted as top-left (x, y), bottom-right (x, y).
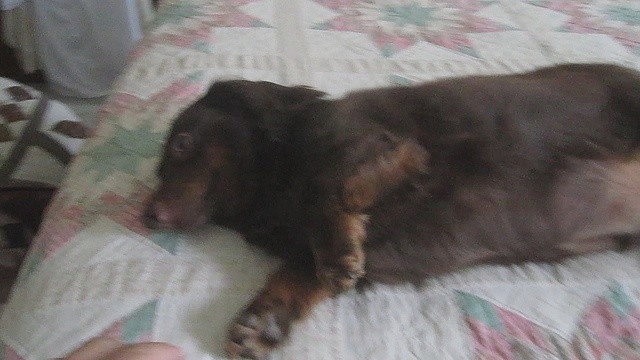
top-left (140, 64), bottom-right (640, 360)
